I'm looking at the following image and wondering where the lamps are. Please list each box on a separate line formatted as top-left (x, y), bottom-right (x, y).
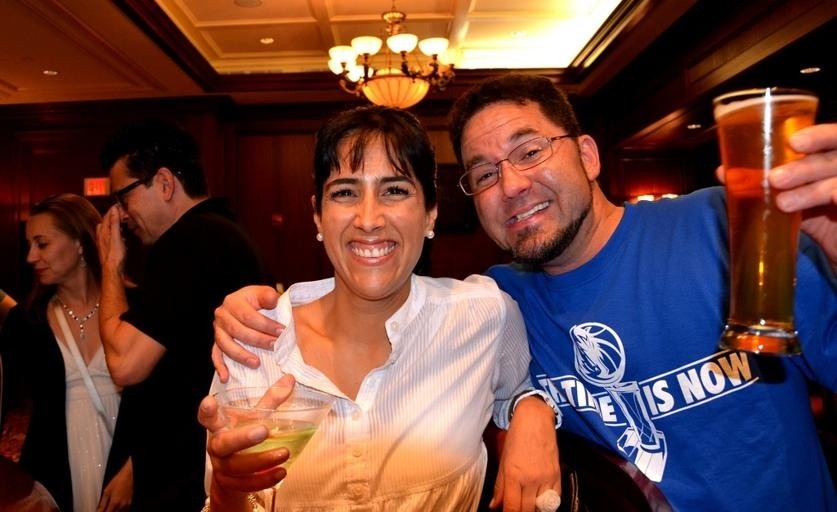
top-left (327, 0), bottom-right (465, 109)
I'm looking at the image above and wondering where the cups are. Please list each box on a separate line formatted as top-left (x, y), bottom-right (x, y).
top-left (713, 88), bottom-right (819, 356)
top-left (211, 386), bottom-right (337, 512)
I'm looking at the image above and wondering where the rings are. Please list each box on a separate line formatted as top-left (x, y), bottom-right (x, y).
top-left (536, 490), bottom-right (562, 512)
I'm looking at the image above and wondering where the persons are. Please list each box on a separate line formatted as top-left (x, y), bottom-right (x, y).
top-left (212, 74), bottom-right (837, 512)
top-left (0, 457), bottom-right (62, 511)
top-left (198, 106), bottom-right (562, 511)
top-left (1, 290), bottom-right (18, 316)
top-left (0, 192), bottom-right (134, 512)
top-left (101, 117), bottom-right (260, 512)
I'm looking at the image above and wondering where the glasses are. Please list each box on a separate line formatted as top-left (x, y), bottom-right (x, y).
top-left (113, 169), bottom-right (181, 207)
top-left (456, 133), bottom-right (576, 196)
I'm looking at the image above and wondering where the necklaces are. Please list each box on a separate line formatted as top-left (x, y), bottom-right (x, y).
top-left (56, 293), bottom-right (101, 340)
